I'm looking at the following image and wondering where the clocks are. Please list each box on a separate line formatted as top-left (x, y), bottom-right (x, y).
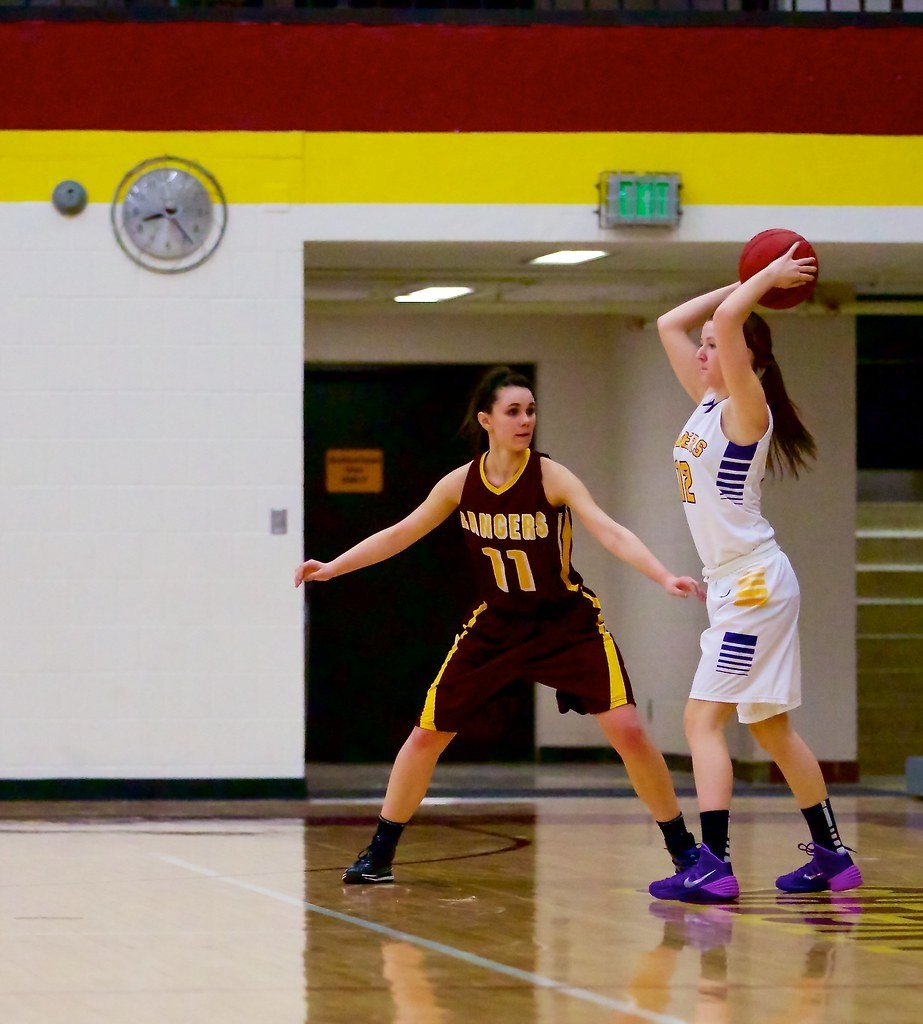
top-left (109, 154), bottom-right (230, 275)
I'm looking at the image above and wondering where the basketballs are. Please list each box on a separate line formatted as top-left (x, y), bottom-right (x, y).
top-left (736, 225), bottom-right (824, 312)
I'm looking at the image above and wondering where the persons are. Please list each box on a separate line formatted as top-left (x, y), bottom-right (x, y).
top-left (294, 366), bottom-right (707, 887)
top-left (647, 241), bottom-right (864, 903)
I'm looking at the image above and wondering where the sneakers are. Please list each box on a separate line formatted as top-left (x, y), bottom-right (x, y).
top-left (775, 842), bottom-right (863, 892)
top-left (342, 844), bottom-right (394, 883)
top-left (649, 843), bottom-right (739, 903)
top-left (667, 847), bottom-right (701, 874)
